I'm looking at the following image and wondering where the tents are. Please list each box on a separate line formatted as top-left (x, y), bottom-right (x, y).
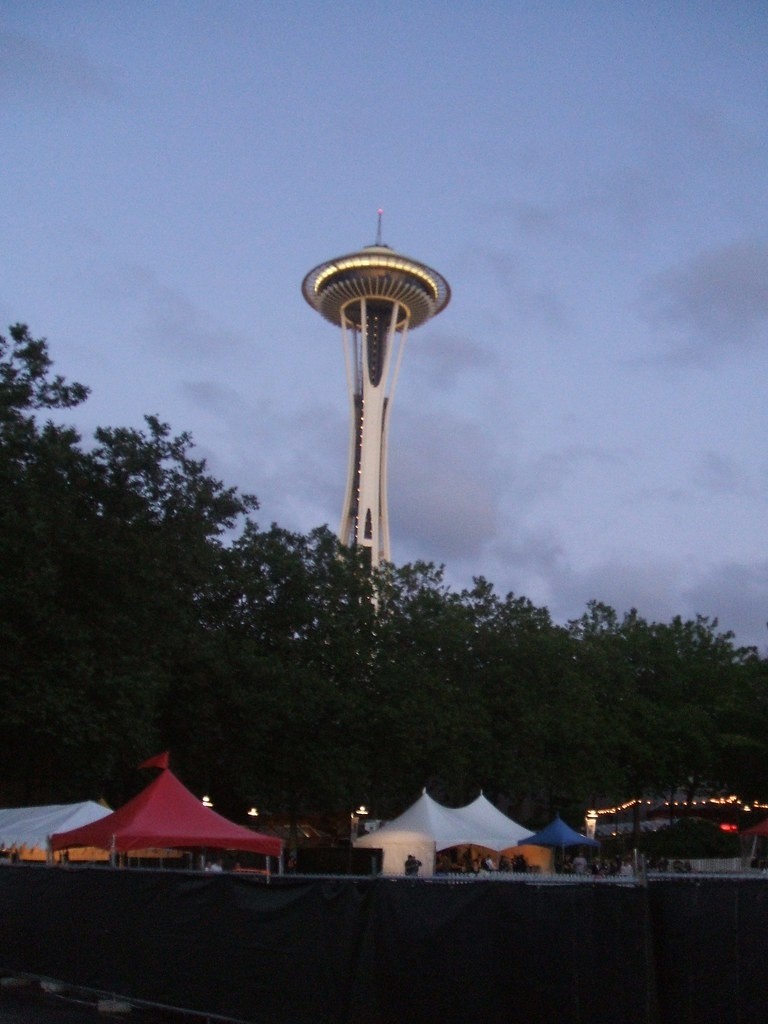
top-left (351, 788), bottom-right (538, 876)
top-left (45, 751), bottom-right (284, 882)
top-left (0, 802), bottom-right (197, 868)
top-left (742, 816), bottom-right (768, 857)
top-left (516, 815), bottom-right (604, 872)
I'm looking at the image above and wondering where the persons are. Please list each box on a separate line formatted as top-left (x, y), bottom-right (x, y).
top-left (404, 854), bottom-right (422, 877)
top-left (204, 857), bottom-right (224, 873)
top-left (437, 847), bottom-right (693, 873)
